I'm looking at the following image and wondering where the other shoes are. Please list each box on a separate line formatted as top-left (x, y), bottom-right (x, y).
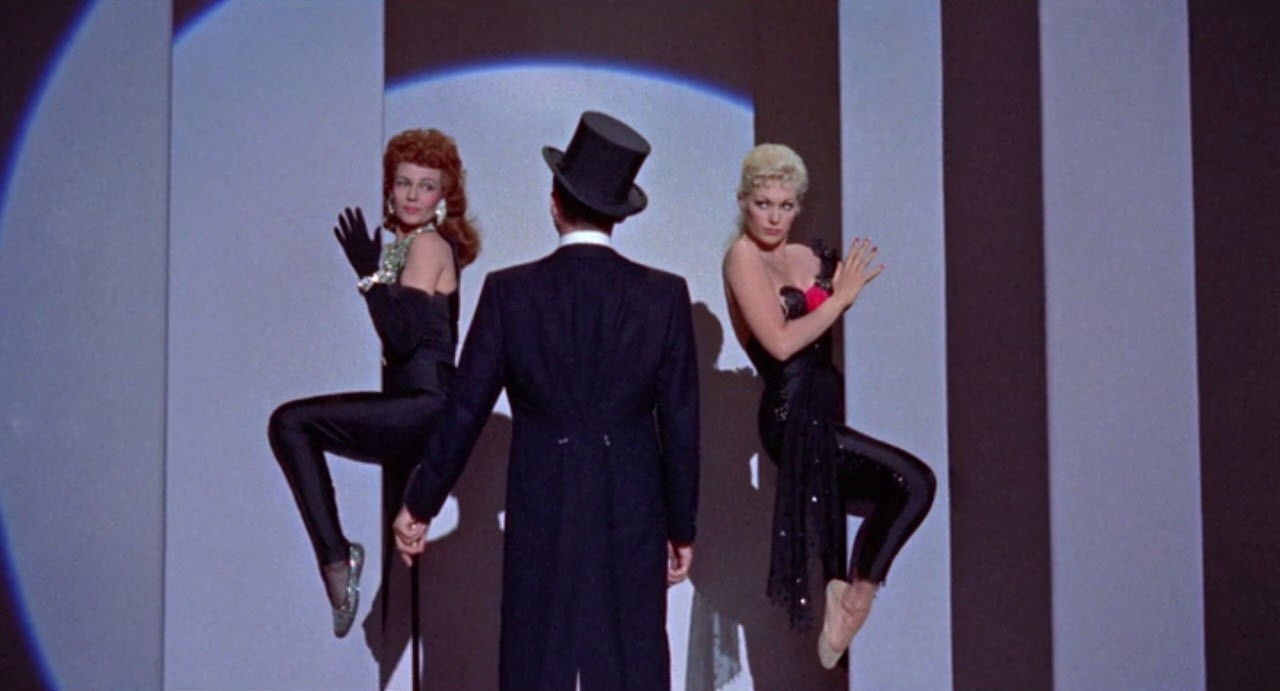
top-left (818, 579), bottom-right (849, 670)
top-left (333, 544), bottom-right (364, 638)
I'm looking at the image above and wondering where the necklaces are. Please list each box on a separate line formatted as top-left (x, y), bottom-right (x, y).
top-left (379, 219), bottom-right (451, 300)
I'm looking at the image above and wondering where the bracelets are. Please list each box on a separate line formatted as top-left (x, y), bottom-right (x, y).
top-left (353, 270), bottom-right (388, 294)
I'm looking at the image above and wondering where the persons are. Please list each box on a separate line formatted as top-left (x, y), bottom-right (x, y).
top-left (266, 128), bottom-right (483, 639)
top-left (721, 143), bottom-right (936, 672)
top-left (393, 112), bottom-right (707, 691)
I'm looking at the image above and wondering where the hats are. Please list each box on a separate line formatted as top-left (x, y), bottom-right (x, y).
top-left (543, 111), bottom-right (651, 215)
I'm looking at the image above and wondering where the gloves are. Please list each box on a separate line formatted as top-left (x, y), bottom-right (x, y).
top-left (334, 207), bottom-right (381, 279)
